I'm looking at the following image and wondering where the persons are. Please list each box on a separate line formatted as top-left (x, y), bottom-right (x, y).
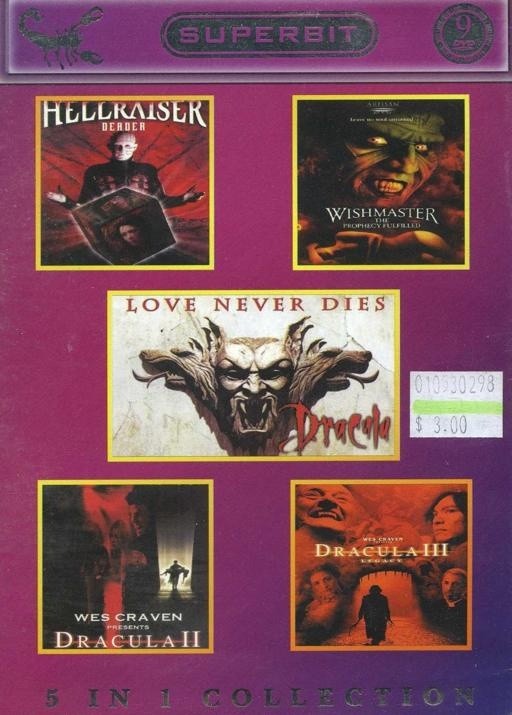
top-left (161, 560), bottom-right (189, 591)
top-left (296, 100), bottom-right (465, 263)
top-left (133, 312), bottom-right (380, 457)
top-left (45, 131), bottom-right (207, 264)
top-left (294, 484), bottom-right (469, 645)
